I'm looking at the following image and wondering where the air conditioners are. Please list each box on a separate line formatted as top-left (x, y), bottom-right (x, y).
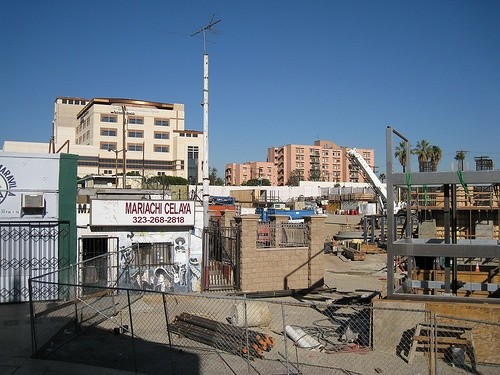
top-left (21, 194), bottom-right (45, 208)
